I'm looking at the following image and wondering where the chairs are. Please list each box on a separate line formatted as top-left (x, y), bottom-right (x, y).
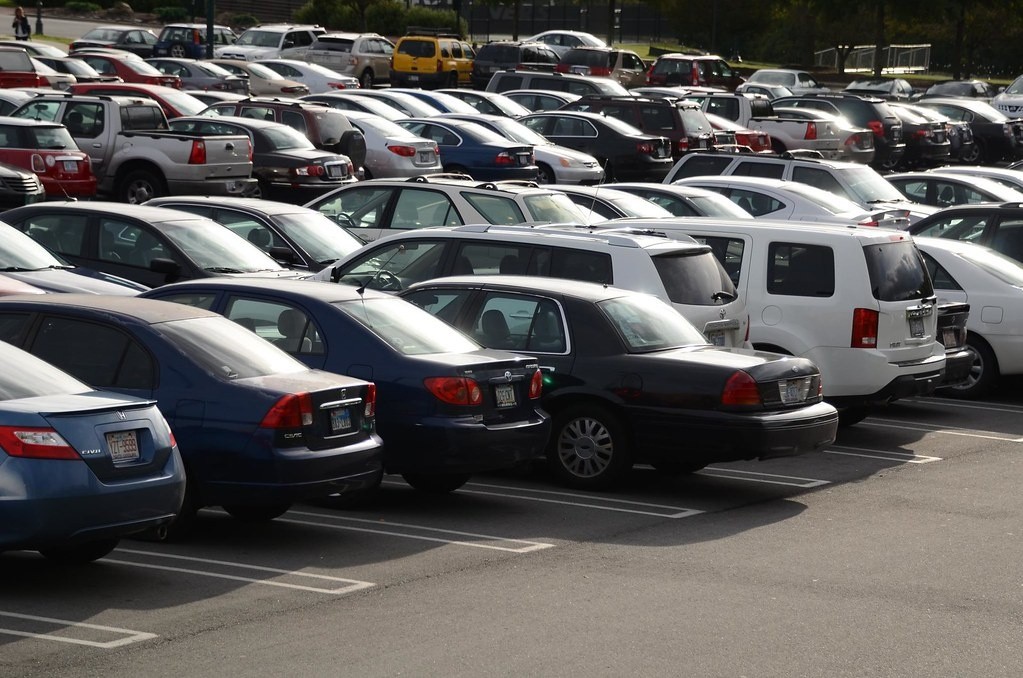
top-left (165, 67), bottom-right (174, 75)
top-left (265, 114), bottom-right (274, 121)
top-left (479, 309), bottom-right (515, 350)
top-left (528, 312), bottom-right (563, 353)
top-left (738, 197), bottom-right (752, 214)
top-left (247, 228), bottom-right (273, 253)
top-left (443, 135), bottom-right (458, 145)
top-left (272, 309), bottom-right (312, 353)
top-left (244, 114), bottom-right (255, 118)
top-left (232, 318), bottom-right (256, 334)
top-left (5, 131), bottom-right (18, 148)
top-left (103, 64), bottom-right (110, 73)
top-left (128, 231), bottom-right (159, 268)
top-left (103, 230), bottom-right (122, 263)
top-left (88, 110), bottom-right (104, 138)
top-left (925, 185), bottom-right (939, 202)
top-left (393, 201), bottom-right (422, 228)
top-left (475, 103), bottom-right (489, 114)
top-left (429, 202), bottom-right (454, 226)
top-left (64, 112), bottom-right (85, 137)
top-left (500, 255), bottom-right (520, 274)
top-left (940, 186), bottom-right (953, 206)
top-left (433, 255), bottom-right (474, 278)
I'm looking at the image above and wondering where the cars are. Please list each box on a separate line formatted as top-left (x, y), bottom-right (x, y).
top-left (0, 23), bottom-right (1022, 572)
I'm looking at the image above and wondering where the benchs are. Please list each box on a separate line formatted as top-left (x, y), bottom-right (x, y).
top-left (35, 229), bottom-right (81, 253)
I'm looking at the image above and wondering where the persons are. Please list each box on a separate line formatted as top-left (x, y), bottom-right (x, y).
top-left (13, 7), bottom-right (31, 40)
